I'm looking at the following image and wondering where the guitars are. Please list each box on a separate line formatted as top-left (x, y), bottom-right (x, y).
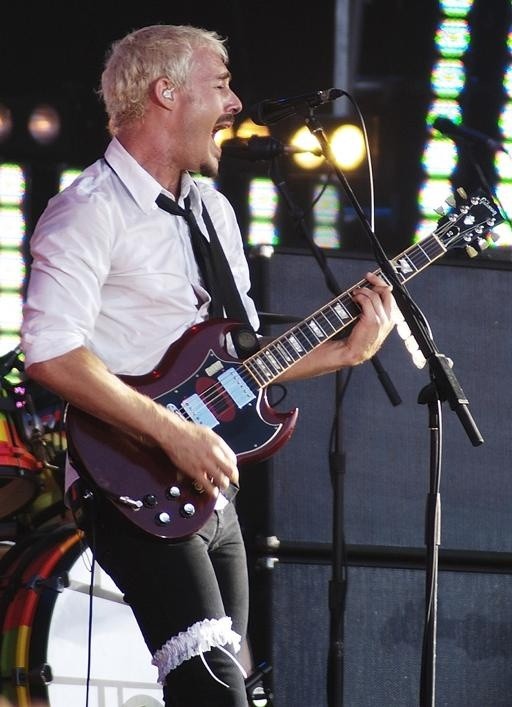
top-left (65, 186), bottom-right (503, 540)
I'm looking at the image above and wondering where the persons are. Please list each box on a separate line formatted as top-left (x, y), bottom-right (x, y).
top-left (18, 23), bottom-right (396, 707)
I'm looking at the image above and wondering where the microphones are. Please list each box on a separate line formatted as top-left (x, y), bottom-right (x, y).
top-left (252, 89), bottom-right (338, 127)
top-left (220, 136), bottom-right (297, 162)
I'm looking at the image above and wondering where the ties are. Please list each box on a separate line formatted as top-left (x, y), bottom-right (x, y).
top-left (156, 192), bottom-right (227, 354)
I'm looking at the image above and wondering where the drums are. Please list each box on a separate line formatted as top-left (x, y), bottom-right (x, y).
top-left (1, 520), bottom-right (167, 706)
top-left (0, 373), bottom-right (47, 523)
